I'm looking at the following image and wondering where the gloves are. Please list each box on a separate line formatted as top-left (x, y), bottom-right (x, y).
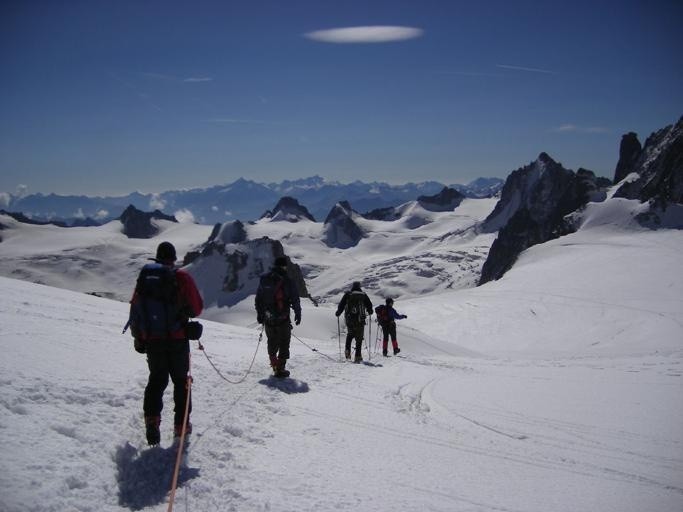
top-left (366, 310), bottom-right (373, 314)
top-left (257, 317), bottom-right (263, 323)
top-left (403, 315), bottom-right (407, 318)
top-left (336, 311), bottom-right (341, 316)
top-left (295, 313), bottom-right (301, 325)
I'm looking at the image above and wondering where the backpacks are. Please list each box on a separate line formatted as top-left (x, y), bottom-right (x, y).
top-left (344, 291), bottom-right (365, 327)
top-left (375, 305), bottom-right (391, 327)
top-left (258, 272), bottom-right (288, 319)
top-left (129, 264), bottom-right (184, 343)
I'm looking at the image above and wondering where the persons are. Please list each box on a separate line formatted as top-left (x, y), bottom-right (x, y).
top-left (254, 256), bottom-right (302, 378)
top-left (128, 240), bottom-right (203, 445)
top-left (382, 297), bottom-right (407, 357)
top-left (335, 281), bottom-right (373, 365)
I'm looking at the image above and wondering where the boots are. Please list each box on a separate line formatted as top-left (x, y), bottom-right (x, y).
top-left (174, 414), bottom-right (192, 436)
top-left (145, 416), bottom-right (160, 445)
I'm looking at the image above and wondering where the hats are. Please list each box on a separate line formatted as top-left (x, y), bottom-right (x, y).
top-left (156, 242), bottom-right (177, 261)
top-left (273, 257), bottom-right (287, 267)
top-left (386, 298), bottom-right (393, 304)
top-left (353, 282), bottom-right (360, 289)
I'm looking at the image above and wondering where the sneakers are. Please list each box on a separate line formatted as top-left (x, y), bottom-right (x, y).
top-left (355, 356), bottom-right (362, 360)
top-left (384, 349), bottom-right (387, 356)
top-left (394, 348), bottom-right (400, 355)
top-left (345, 350), bottom-right (351, 359)
top-left (272, 364), bottom-right (289, 377)
